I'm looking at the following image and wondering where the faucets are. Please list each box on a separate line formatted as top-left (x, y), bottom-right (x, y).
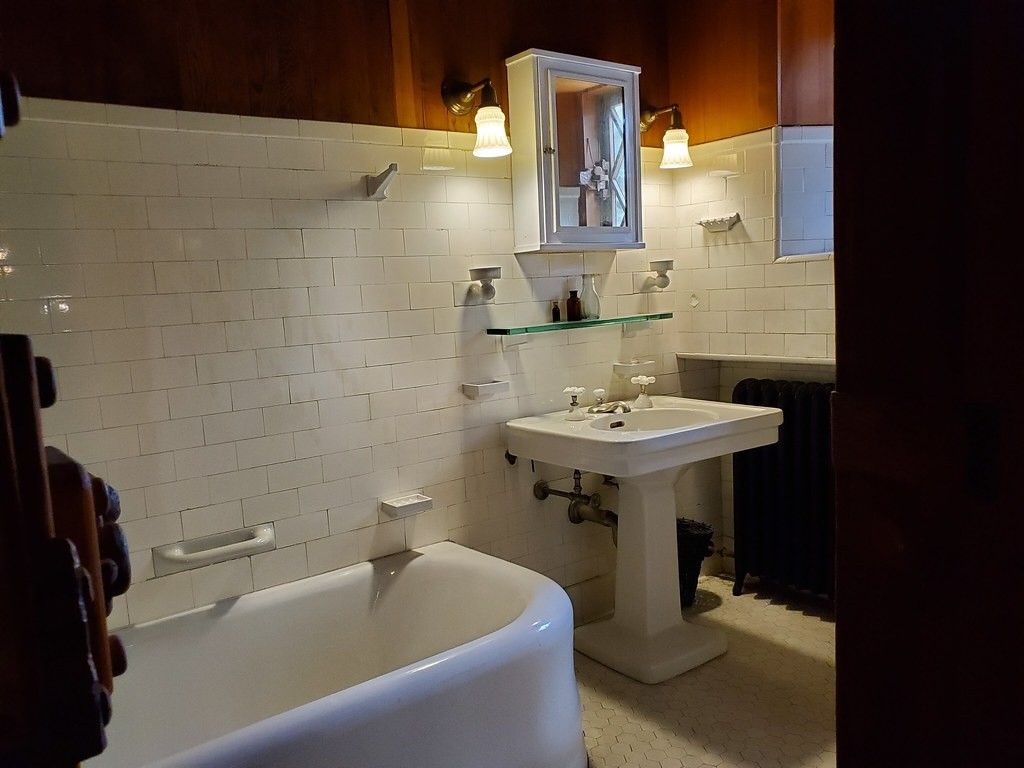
top-left (587, 388), bottom-right (631, 414)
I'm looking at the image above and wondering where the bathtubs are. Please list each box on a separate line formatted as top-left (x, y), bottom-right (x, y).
top-left (79, 539), bottom-right (591, 768)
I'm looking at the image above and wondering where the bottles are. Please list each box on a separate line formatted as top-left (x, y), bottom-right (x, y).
top-left (552, 302), bottom-right (560, 323)
top-left (566, 290), bottom-right (582, 321)
top-left (580, 277), bottom-right (600, 318)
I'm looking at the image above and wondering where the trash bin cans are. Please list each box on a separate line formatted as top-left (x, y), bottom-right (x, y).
top-left (674, 518), bottom-right (714, 607)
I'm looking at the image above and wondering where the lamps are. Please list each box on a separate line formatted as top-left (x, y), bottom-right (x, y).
top-left (640, 103), bottom-right (693, 168)
top-left (442, 78), bottom-right (513, 158)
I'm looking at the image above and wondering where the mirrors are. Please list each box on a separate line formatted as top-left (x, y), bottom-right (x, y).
top-left (505, 48), bottom-right (646, 253)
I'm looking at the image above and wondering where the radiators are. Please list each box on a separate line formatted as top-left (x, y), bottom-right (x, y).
top-left (732, 378), bottom-right (836, 623)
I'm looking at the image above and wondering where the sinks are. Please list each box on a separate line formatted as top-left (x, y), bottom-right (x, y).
top-left (505, 394), bottom-right (783, 478)
